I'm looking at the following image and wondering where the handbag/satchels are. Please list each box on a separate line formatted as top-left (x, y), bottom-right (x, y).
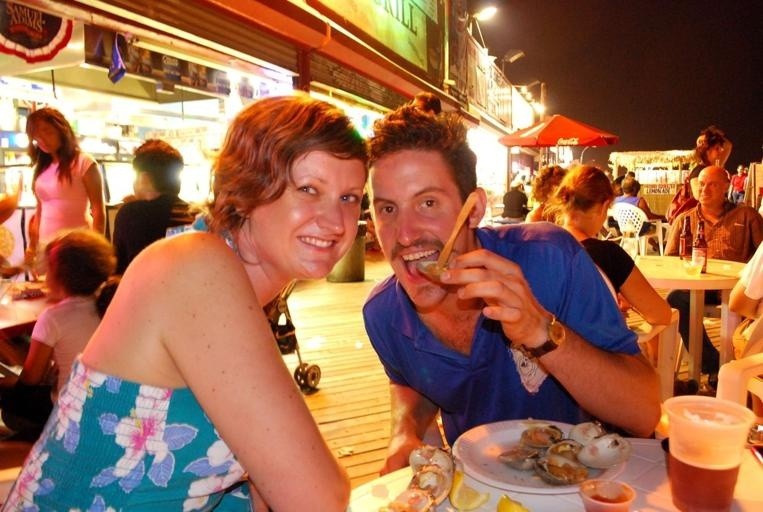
top-left (664, 177), bottom-right (698, 225)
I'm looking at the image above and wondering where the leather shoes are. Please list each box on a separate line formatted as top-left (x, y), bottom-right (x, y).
top-left (708, 374), bottom-right (716, 385)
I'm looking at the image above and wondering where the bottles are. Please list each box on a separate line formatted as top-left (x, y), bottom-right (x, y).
top-left (649, 186), bottom-right (670, 193)
top-left (679, 215), bottom-right (693, 261)
top-left (637, 154), bottom-right (692, 171)
top-left (691, 220), bottom-right (709, 274)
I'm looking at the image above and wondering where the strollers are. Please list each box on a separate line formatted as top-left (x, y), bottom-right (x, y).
top-left (262, 277), bottom-right (321, 390)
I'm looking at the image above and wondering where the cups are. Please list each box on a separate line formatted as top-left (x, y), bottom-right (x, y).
top-left (683, 256), bottom-right (707, 277)
top-left (0, 279), bottom-right (12, 302)
top-left (662, 395), bottom-right (758, 512)
top-left (577, 478), bottom-right (636, 512)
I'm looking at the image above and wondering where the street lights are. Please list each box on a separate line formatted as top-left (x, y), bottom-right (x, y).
top-left (579, 143), bottom-right (596, 167)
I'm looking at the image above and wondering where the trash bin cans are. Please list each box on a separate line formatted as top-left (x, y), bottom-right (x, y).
top-left (327, 220), bottom-right (367, 282)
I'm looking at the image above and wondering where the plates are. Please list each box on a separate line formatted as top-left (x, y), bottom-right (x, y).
top-left (450, 419), bottom-right (627, 497)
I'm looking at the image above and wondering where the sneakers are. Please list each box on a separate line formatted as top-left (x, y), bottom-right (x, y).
top-left (674, 377), bottom-right (698, 395)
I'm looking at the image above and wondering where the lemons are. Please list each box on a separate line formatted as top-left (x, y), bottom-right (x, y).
top-left (496, 494), bottom-right (529, 512)
top-left (449, 472), bottom-right (488, 510)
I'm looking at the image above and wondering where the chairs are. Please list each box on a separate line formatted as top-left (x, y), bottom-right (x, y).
top-left (599, 199), bottom-right (762, 435)
